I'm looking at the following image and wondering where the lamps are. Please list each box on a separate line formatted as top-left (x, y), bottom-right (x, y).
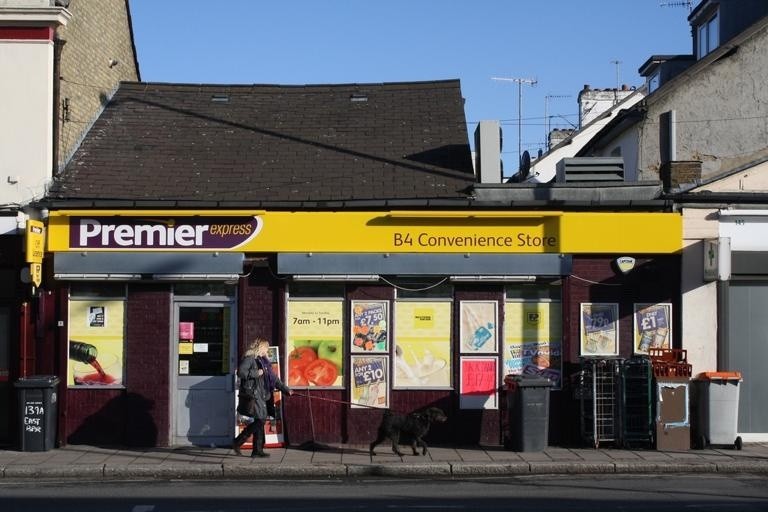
top-left (109, 58), bottom-right (118, 69)
top-left (62, 97), bottom-right (71, 123)
top-left (615, 256), bottom-right (636, 277)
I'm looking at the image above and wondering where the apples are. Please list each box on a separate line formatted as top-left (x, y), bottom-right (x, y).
top-left (294, 340), bottom-right (320, 353)
top-left (317, 340), bottom-right (343, 376)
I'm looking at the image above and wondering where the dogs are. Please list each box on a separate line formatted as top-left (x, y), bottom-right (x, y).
top-left (369, 407), bottom-right (448, 456)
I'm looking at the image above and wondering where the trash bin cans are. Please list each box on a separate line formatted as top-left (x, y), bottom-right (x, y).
top-left (692, 371), bottom-right (742, 450)
top-left (13, 374), bottom-right (63, 452)
top-left (505, 374), bottom-right (553, 452)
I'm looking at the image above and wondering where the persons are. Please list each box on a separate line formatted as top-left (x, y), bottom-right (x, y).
top-left (232, 336), bottom-right (296, 459)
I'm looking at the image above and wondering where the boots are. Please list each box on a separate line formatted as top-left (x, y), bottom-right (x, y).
top-left (233, 430), bottom-right (249, 455)
top-left (251, 439), bottom-right (270, 457)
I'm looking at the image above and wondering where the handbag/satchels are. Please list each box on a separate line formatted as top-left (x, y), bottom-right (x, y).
top-left (237, 394), bottom-right (256, 417)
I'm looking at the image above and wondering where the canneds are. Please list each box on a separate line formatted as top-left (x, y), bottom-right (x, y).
top-left (651, 327), bottom-right (668, 349)
top-left (638, 331), bottom-right (654, 353)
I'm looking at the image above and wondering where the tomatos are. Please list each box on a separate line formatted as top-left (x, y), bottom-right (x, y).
top-left (287, 346), bottom-right (338, 386)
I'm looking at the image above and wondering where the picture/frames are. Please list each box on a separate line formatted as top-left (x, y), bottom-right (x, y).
top-left (632, 303), bottom-right (673, 356)
top-left (580, 302), bottom-right (620, 357)
top-left (459, 300), bottom-right (500, 355)
top-left (350, 355), bottom-right (389, 409)
top-left (460, 356), bottom-right (500, 410)
top-left (502, 299), bottom-right (564, 392)
top-left (66, 296), bottom-right (125, 391)
top-left (392, 299), bottom-right (454, 390)
top-left (283, 291), bottom-right (346, 390)
top-left (351, 300), bottom-right (390, 354)
